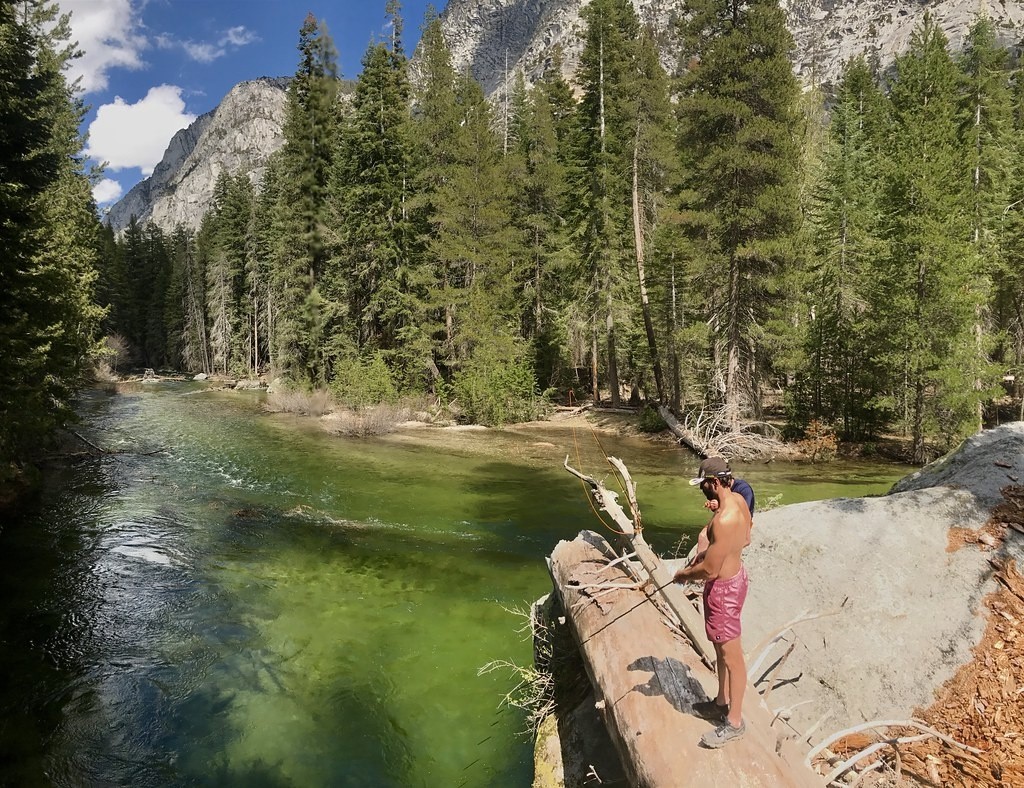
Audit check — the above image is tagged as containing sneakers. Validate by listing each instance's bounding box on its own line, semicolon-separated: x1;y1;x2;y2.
683;579;703;595
691;579;706;596
701;716;746;748
692;696;731;719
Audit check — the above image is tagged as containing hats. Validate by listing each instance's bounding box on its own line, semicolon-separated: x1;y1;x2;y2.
688;457;732;486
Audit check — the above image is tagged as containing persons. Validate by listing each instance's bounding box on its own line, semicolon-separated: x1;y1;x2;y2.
673;456;755;749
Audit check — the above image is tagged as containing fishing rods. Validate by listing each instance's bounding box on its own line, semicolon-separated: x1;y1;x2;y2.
567;387;644;536
499;563;674;692
